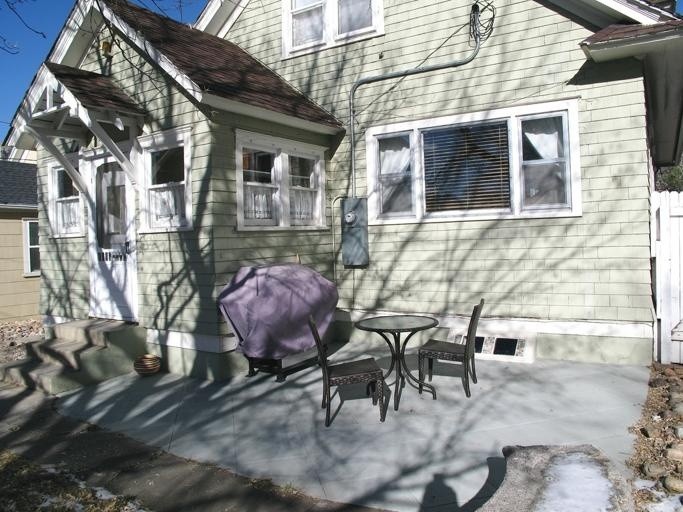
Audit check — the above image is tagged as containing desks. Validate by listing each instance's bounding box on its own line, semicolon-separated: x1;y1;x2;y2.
354;315;439;411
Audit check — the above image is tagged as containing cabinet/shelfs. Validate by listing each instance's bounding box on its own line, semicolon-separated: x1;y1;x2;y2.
247;345;328;382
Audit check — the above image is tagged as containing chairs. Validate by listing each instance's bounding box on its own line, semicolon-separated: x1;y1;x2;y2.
308;314;385;427
419;298;485;398
